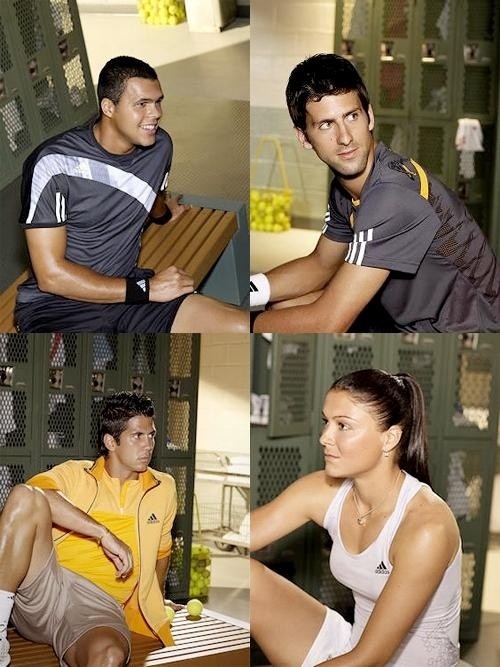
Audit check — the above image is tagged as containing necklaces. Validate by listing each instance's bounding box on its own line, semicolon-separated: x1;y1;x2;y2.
353;468;403;526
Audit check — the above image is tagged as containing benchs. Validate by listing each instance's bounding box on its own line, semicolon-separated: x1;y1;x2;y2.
0;605;250;667
0;191;250;332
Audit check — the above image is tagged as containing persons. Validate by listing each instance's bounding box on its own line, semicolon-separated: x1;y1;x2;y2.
251;367;462;666
13;57;249;332
0;392;179;667
250;51;500;334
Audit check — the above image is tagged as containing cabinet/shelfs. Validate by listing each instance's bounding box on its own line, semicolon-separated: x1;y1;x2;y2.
0;0;100;190
249;333;500;666
330;0;499;262
0;332;200;602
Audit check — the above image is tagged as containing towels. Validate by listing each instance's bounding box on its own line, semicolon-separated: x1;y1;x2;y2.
455;118;484;179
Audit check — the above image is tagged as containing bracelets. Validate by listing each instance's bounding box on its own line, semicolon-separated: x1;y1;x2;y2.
149;203;173;225
124;276;150;305
249;272;271;307
96;528;111;544
250;309;264;334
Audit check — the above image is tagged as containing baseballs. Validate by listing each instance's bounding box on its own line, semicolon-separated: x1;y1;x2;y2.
188;546;212;599
165;605;173;625
136;1;185;27
250;189;292;233
185;600;204;617
